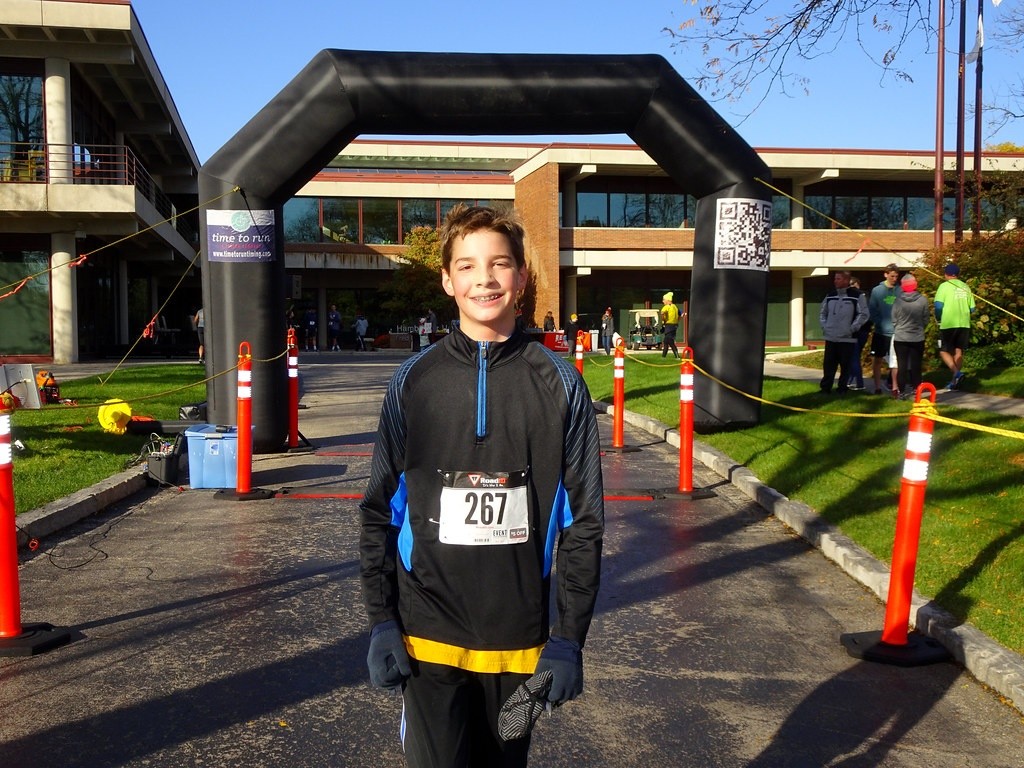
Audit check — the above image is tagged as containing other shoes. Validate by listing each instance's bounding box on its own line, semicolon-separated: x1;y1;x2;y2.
819;388;832;394
874;388;883;394
900;391;906;399
892;388;901;399
305;349;310;352
338;349;342;352
836;386;848;394
314;349;319;353
911;389;917;395
850;384;866;391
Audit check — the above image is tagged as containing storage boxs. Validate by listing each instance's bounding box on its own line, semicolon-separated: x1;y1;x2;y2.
147;426;189;486
184;424;256;489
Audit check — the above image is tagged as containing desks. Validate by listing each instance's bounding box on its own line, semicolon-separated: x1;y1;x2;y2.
434;333;448;336
540;333;569;351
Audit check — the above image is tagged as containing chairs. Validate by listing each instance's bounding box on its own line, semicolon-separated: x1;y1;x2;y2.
28;149;46;181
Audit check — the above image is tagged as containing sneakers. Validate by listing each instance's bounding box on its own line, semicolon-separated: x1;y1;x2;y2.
946;383;960;392
954;371;966;390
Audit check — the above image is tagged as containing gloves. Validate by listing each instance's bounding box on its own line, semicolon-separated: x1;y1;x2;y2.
367;621;411;688
532;639;584;710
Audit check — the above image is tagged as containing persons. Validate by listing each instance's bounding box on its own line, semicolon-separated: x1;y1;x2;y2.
564;313;580;357
155;305;205;364
418;307;437;354
543;311;558;332
285;304;369;352
359;202;605;768
602;307;614;357
660;292;682;357
934;264;976;392
819;263;928;400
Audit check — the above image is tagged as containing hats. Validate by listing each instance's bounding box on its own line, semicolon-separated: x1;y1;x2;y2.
945;263;960;277
901;274;917;292
663;292;673;302
419;317;425;323
570;313;577;320
606;307;612;315
499;669;554;739
